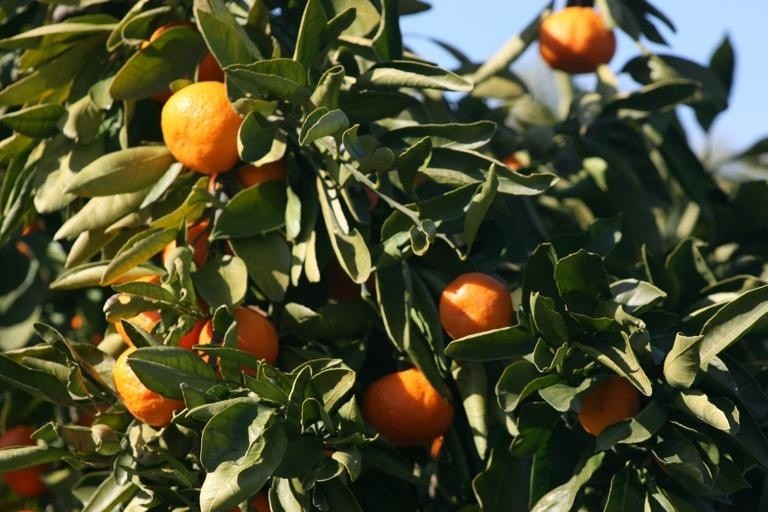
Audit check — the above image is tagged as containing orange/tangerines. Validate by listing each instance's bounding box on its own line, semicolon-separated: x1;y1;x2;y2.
0;20;522;512
576;378;640;436
539;6;616;74
